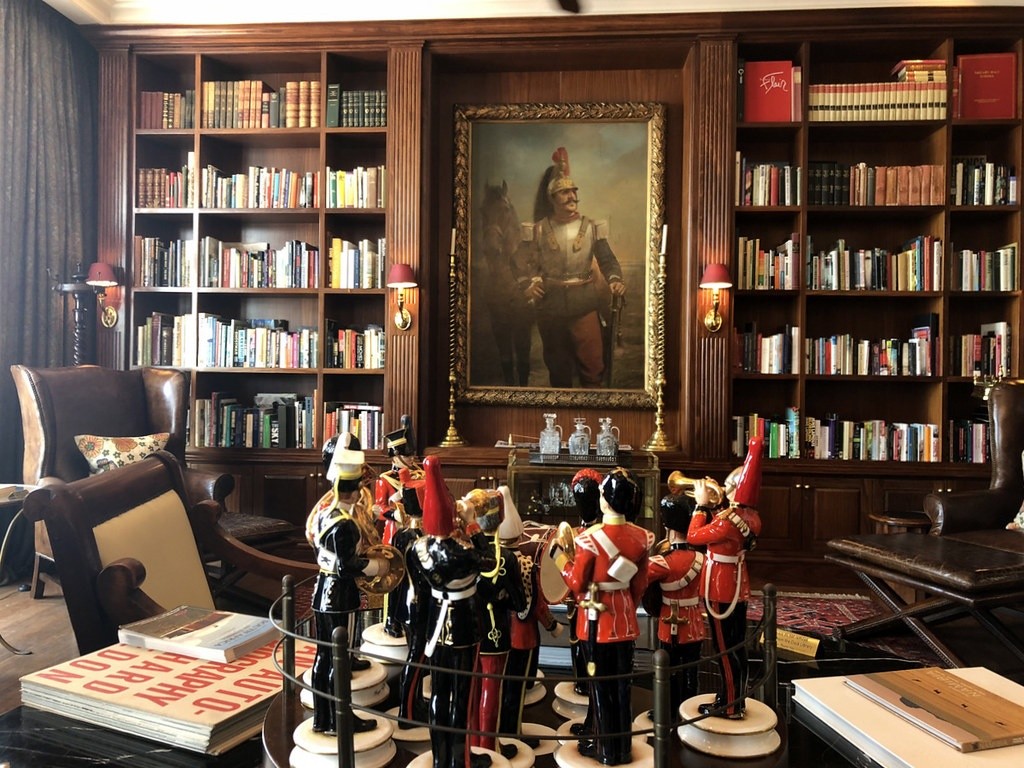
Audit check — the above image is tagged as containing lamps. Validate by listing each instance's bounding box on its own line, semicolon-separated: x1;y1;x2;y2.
87;263;120;330
698;262;734;334
386;262;418;330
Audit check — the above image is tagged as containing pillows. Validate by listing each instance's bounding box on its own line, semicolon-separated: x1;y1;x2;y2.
1006;448;1024;533
68;431;175;478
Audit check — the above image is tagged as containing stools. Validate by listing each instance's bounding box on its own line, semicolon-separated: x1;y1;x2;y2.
184;508;303;606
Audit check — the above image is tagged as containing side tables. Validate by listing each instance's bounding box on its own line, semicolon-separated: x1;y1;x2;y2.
868;510;934;613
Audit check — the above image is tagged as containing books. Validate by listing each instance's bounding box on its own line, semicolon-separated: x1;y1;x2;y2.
791;666;1024;768
135;73;386;452
731;49;1017;467
843;666;1024;754
118;604;284;663
20;633;317;755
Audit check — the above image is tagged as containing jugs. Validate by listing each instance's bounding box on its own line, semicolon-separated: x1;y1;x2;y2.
594;416;621;462
538;415;563;460
567;417;591;461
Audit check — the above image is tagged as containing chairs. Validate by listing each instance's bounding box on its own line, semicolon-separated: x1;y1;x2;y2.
11;359;236;601
925;376;1019;624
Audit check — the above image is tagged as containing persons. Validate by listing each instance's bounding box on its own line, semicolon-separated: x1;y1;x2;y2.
304;429;760;767
519;147;626;388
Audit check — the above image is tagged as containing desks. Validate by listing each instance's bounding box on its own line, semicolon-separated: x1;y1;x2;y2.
823;527;1022;670
0;650;1021;768
1;483;42;658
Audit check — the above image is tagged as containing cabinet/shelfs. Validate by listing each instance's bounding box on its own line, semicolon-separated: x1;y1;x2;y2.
185;459;252;518
729;28;1022;462
125;43;392;454
748;473;870;555
253;461;381;521
409;465;509;514
873;474;988;525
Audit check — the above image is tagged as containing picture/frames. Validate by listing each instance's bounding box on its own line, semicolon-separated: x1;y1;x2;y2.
453;96;671;409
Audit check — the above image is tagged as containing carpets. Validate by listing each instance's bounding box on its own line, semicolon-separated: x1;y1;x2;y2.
745;586;951;671
